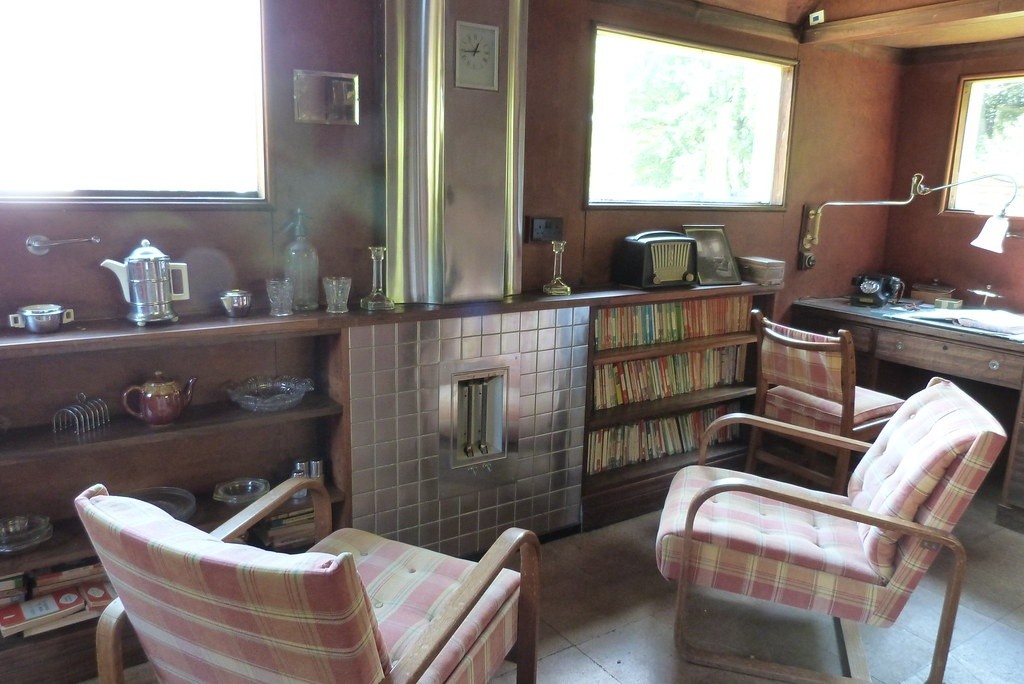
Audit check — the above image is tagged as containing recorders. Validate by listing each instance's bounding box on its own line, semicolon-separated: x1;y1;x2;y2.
620;231;698;290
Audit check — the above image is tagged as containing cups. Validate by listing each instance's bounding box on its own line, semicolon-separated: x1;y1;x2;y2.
323;277;351;313
265;277;294;316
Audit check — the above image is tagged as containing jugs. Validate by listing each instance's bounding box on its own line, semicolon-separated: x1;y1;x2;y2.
100;239;190;326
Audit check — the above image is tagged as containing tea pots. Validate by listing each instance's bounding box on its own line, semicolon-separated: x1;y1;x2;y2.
121;370;198;427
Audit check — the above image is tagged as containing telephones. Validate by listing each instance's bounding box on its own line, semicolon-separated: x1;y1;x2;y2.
851;273;900;308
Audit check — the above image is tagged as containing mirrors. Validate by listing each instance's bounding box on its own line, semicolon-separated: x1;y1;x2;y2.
293;69;360;125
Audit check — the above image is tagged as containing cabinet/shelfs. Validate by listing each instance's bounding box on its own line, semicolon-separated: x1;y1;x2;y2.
0;311;345;684
582;280;781;531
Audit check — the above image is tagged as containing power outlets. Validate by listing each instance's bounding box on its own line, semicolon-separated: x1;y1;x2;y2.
527;216;565;244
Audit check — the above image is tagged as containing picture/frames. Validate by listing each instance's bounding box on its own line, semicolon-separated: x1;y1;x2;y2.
680;223;742;287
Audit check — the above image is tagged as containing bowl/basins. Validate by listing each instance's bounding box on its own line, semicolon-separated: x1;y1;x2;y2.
0;514;53;555
225;375;314;411
212;477;270;511
116;487;196;522
218;288;252;318
9;304;74;332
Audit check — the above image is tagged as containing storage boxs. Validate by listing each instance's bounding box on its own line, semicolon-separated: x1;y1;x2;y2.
736;255;785;285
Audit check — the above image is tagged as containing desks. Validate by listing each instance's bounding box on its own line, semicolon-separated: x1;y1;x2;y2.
792;294;1024;537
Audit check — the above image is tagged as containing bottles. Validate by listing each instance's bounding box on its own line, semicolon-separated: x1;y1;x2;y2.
289;456;324;498
283;207;319;310
931;279;939;287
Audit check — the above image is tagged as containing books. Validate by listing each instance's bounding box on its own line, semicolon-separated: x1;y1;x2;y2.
587;295;752;474
0;561;118;638
915;308;1024;335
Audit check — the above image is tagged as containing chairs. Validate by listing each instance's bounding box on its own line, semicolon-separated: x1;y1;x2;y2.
75;474;541;683
655;376;1009;684
741;309;906;496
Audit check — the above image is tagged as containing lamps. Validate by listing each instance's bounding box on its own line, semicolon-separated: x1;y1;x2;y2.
796;173;1018;272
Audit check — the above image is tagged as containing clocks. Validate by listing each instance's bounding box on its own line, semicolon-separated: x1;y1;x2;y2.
454;20;502;92
